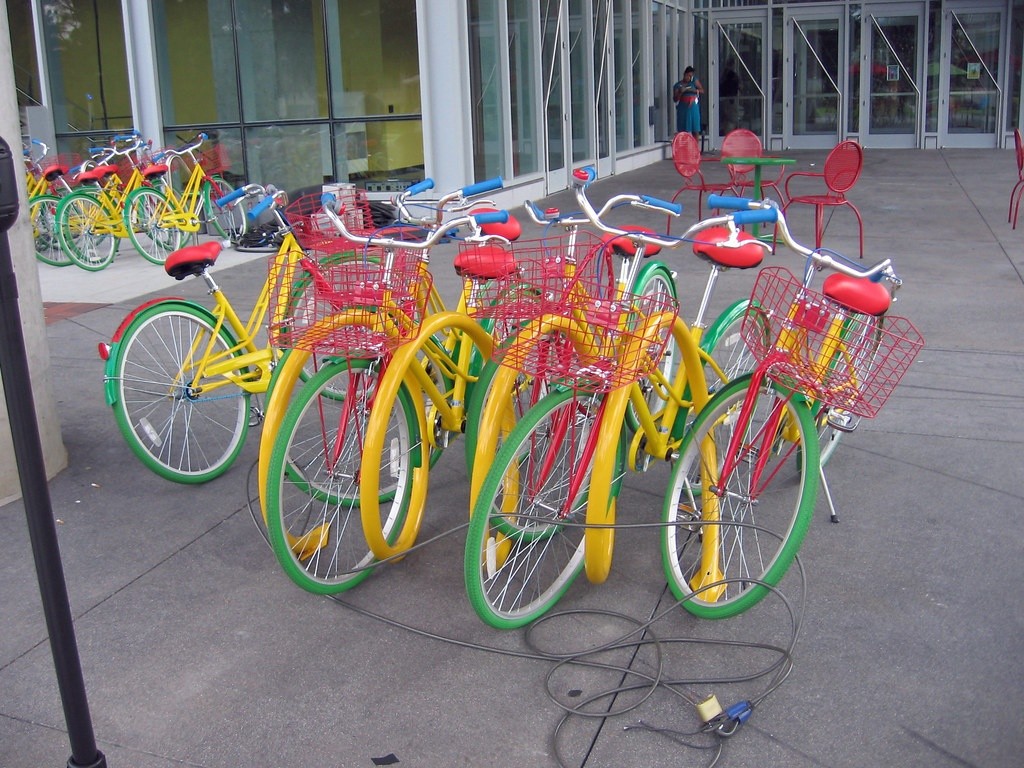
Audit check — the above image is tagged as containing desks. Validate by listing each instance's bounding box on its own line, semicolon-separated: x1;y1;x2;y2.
720;156;796;244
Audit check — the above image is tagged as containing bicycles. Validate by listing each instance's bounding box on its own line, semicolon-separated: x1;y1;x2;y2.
98;184;417;485
660;194;923;615
252;164;781;633
22;131;251;271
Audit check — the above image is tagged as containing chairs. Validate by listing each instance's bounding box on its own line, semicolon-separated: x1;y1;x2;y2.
667;131;745;236
716;129;788;232
771;141;863;265
1008;128;1024;230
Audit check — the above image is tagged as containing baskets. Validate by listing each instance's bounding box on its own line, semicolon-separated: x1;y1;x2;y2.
42;153;82;187
458;229;680;394
267;190;433;359
99;145;180;186
183;143;231;176
740;267;926;418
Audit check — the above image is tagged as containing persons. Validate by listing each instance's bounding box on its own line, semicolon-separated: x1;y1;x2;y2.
673;66;705;144
719;57;753;136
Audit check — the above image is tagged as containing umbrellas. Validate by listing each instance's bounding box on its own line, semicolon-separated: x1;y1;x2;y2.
927;62;968;76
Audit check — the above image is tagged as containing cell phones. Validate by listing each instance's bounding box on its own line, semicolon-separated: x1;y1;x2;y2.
691;76;693;82
